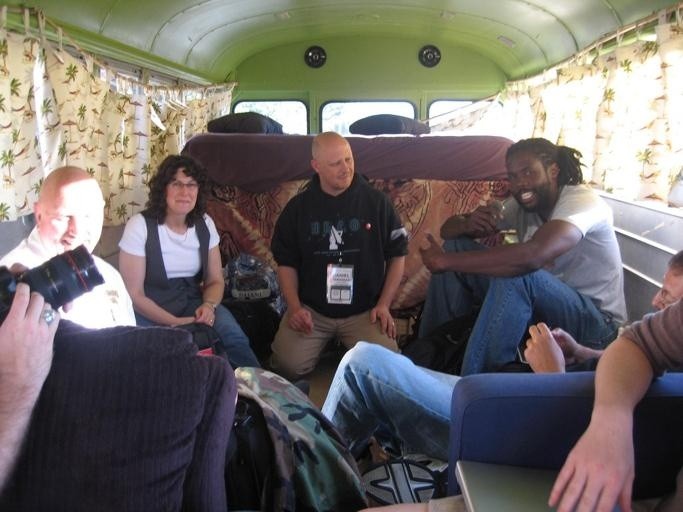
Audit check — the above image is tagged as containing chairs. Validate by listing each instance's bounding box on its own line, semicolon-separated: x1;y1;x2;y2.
1;318;240;512
447;372;682;497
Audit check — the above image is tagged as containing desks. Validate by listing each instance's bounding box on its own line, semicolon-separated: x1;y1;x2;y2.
453;460;662;512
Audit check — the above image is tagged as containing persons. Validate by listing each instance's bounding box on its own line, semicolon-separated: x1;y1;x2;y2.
398;137;629;377
1;262;73;493
117;154;261;369
321;250;683;463
261;130;409;377
1;165;137;330
547;299;683;512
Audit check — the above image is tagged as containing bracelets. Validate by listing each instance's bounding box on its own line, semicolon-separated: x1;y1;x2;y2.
202;301;216;312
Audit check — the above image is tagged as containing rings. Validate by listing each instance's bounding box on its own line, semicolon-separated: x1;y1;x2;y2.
392;321;395;326
44;309;55;324
210;319;213;323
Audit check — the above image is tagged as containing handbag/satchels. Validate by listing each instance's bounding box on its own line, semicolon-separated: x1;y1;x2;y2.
223;299;280;361
224;367;368;510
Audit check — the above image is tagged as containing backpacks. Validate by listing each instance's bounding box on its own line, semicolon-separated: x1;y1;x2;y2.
222;254;281;314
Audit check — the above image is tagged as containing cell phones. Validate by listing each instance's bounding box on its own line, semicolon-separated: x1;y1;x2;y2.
490;200;505;220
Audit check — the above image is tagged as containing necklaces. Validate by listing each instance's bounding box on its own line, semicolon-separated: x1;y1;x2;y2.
165;219;188;242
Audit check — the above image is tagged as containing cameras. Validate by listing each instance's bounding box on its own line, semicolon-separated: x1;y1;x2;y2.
0;243;106;326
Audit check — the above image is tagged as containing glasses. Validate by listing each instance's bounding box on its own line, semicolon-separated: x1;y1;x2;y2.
173;180;200;191
